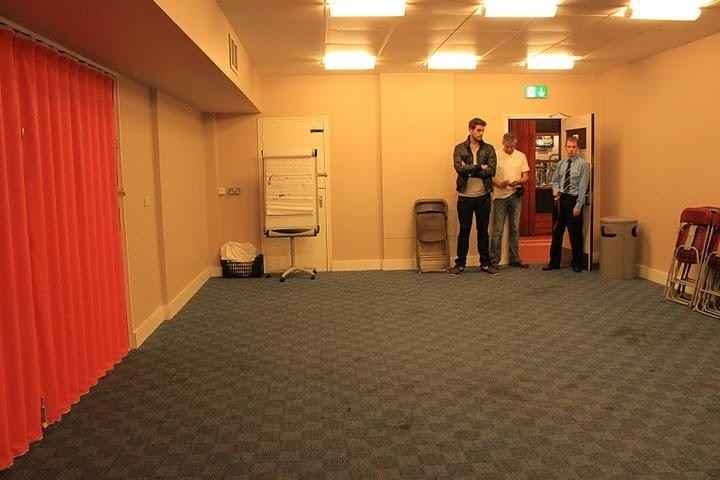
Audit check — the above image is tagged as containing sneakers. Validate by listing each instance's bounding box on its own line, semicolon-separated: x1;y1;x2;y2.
449;266;465;275
571;264;581;272
508;261;528;268
542;264;560;271
481;263;500;274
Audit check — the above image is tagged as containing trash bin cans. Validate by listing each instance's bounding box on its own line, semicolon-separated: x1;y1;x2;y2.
600;217;638;280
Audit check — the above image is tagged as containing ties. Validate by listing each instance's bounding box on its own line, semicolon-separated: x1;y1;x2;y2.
563;160;572;193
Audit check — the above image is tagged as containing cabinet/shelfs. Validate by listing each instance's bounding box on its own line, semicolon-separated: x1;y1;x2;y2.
507;117;560;235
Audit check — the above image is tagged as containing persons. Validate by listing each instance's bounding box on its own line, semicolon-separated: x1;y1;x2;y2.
490;134;530;268
449;118;497;274
542;135;590;272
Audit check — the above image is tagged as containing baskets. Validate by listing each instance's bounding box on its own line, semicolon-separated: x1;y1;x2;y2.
221;254;263;279
